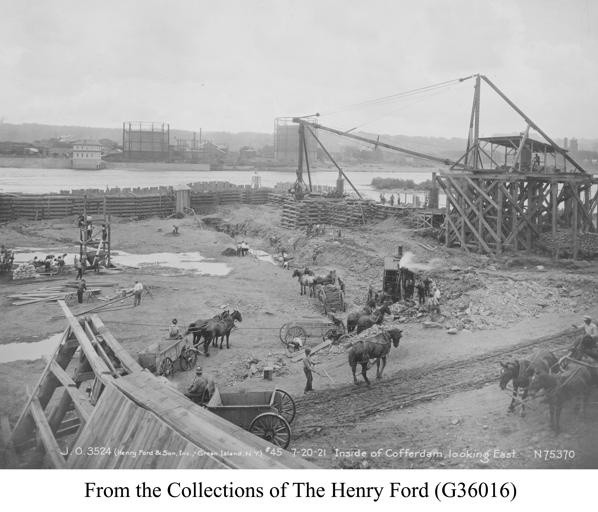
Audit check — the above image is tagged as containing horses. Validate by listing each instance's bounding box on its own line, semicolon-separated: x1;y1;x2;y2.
498;349;559;418
527;364;593;435
348;327;403;386
185;309;230;350
292;268;316;298
357;302;392;335
347;302;376;332
202;310;243;357
303;267;323;292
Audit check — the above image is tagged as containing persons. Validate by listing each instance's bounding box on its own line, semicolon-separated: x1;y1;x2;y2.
571;315;598;350
416;281;426;304
379;193;400;206
237;240;248;257
74;213;107;280
133;280;143;307
184;366;208;394
367;294;378;309
423;276;432;296
302;347;321;394
0;245;7;263
169;318;181;338
33;256;65;273
77;279;88;303
533;154;540;166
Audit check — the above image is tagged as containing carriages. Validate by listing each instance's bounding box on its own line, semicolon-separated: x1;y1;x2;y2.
279;288;393;350
140;303;242;377
292;264;348;317
494;332;596;436
